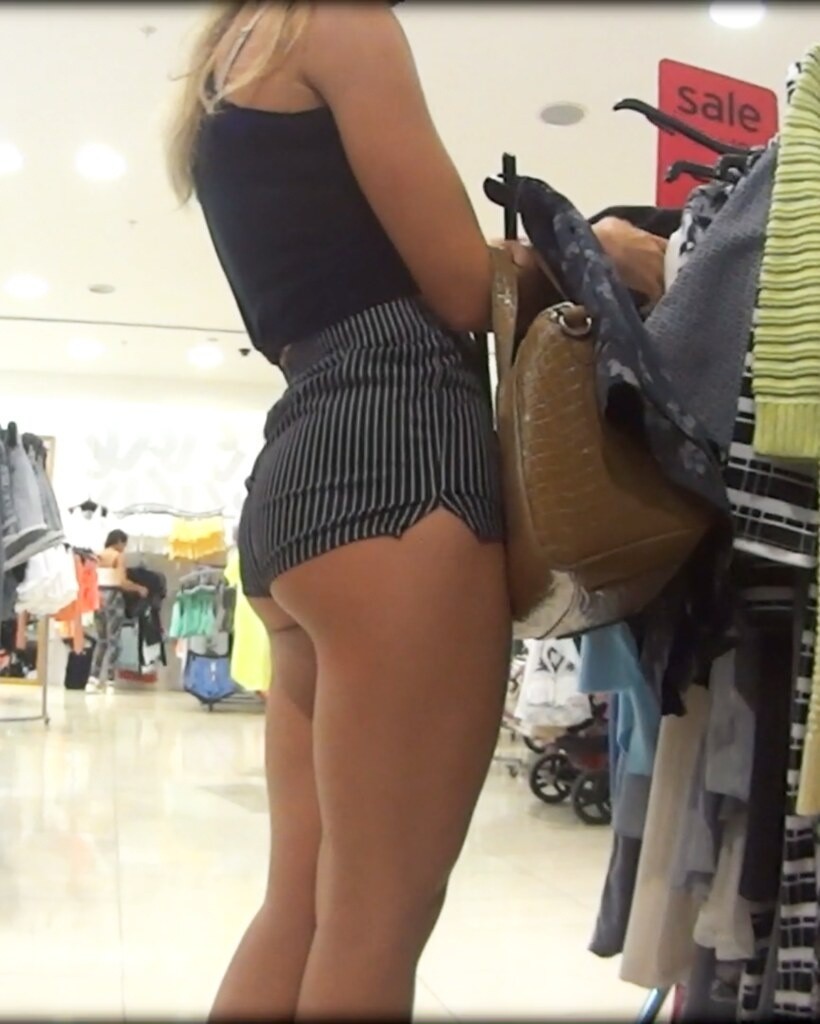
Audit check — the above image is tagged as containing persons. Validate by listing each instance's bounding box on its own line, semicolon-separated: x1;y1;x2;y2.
84;529;149;693
156;2;663;1024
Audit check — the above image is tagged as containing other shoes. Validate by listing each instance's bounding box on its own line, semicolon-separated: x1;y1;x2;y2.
84;676;103;693
106;680;115;694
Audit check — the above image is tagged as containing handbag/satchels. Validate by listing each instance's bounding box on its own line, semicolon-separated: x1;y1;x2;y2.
487;236;704;638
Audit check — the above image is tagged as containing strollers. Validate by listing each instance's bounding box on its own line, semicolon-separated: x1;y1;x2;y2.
526;631;619;827
